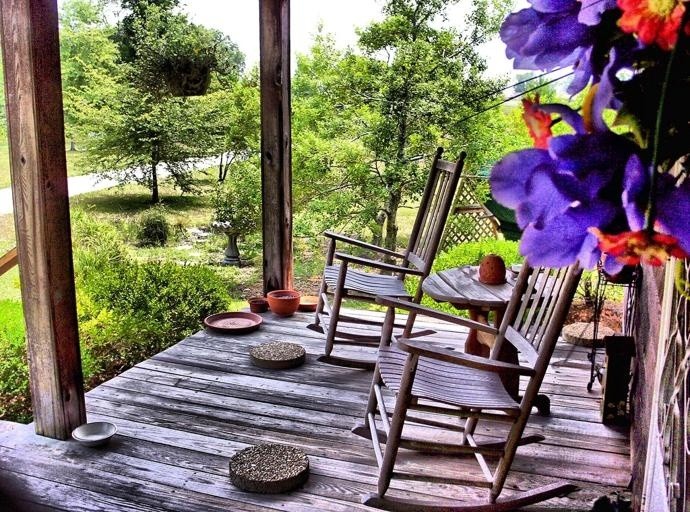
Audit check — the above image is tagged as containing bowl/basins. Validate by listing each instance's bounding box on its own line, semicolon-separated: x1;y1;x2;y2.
298;295;319;311
70;420;118;446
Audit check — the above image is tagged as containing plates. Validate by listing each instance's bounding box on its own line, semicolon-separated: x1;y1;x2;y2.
204;311;262;330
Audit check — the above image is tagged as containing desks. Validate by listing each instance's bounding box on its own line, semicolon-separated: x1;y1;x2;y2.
421;265;559;419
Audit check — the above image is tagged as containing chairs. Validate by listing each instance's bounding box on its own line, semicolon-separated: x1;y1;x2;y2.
305;147;467;370
351;262;584;512
